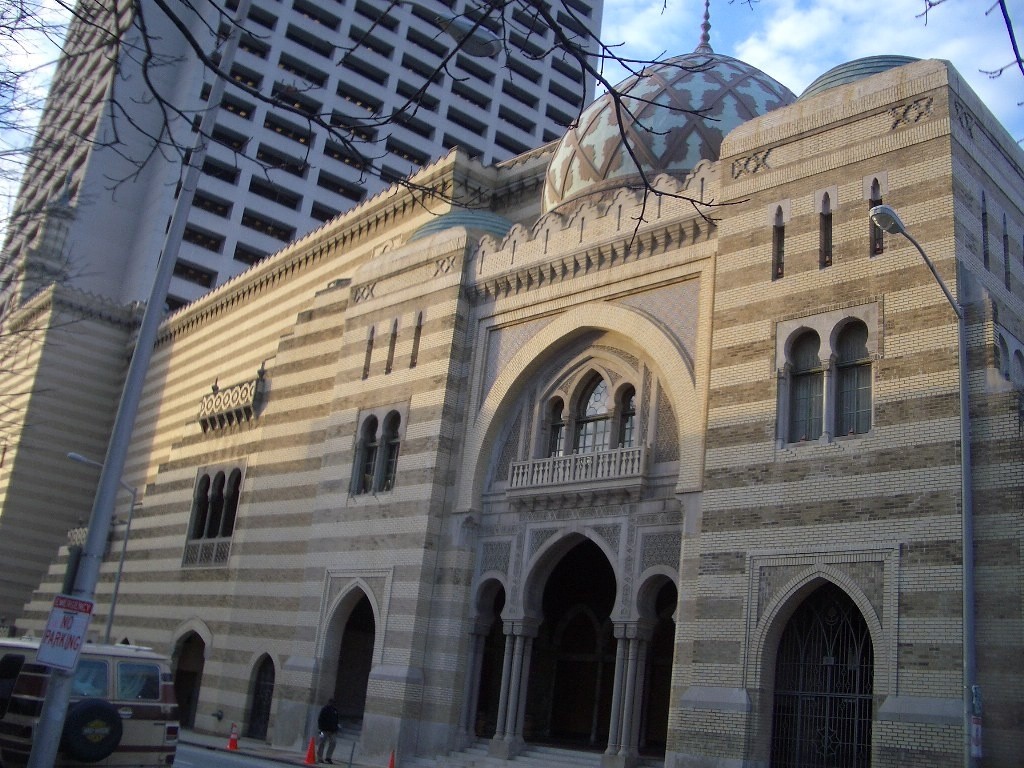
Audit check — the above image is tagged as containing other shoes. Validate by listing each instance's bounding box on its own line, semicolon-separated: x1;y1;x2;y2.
325;758;333;764
318;760;324;763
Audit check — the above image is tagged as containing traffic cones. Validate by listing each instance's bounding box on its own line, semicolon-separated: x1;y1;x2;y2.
386;749;395;768
305;737;316;765
225;722;238;750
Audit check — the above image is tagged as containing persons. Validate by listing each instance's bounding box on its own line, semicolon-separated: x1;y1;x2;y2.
316;699;343;765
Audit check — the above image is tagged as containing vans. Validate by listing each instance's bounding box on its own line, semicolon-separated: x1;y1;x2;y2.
0;632;181;768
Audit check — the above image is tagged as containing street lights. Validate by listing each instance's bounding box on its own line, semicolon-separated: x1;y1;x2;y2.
66;451;142;644
868;204;986;768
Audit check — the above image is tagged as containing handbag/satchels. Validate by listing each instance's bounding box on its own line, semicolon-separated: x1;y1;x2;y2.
319;731;324;739
